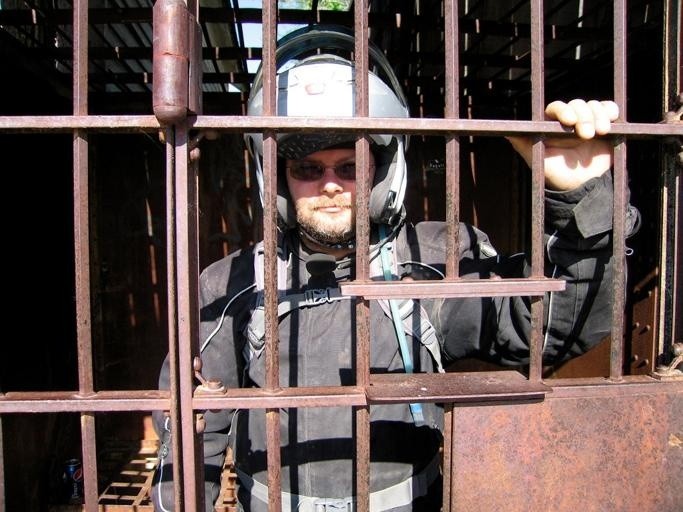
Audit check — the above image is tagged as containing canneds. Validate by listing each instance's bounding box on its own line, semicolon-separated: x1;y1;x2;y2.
62;457;82;500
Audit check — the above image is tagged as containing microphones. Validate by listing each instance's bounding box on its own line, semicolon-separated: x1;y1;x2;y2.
306;203;407;277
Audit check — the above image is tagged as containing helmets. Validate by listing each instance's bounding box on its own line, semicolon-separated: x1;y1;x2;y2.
243;53;412;233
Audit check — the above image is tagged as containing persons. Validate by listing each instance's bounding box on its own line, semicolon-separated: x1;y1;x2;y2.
148;25;641;511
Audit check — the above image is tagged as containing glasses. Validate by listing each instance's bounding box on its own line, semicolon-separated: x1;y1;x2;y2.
285;157;353;181
